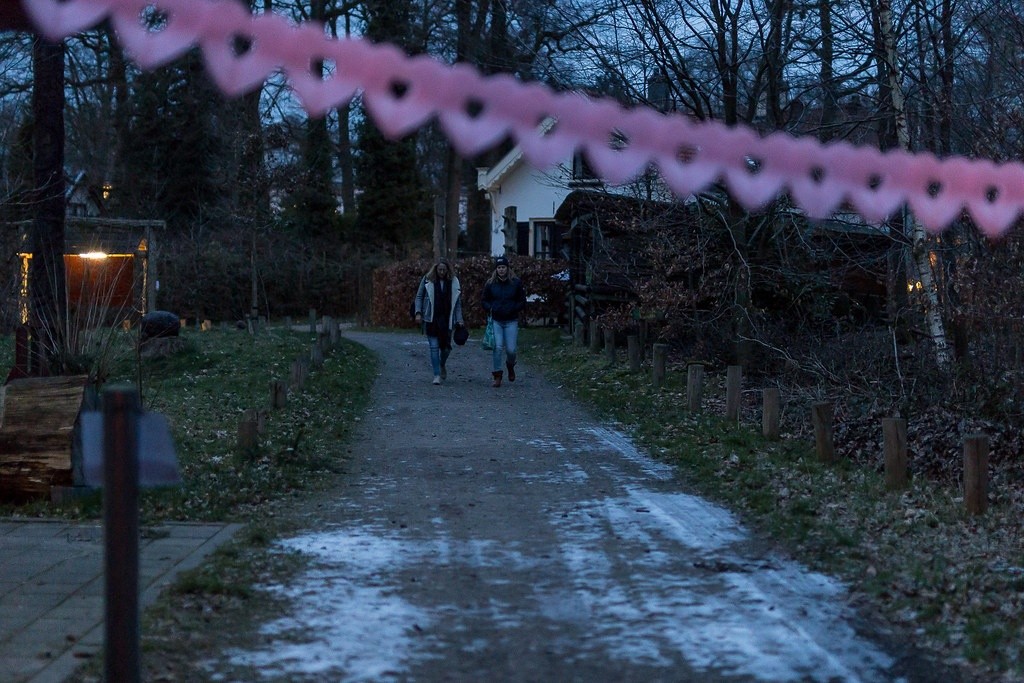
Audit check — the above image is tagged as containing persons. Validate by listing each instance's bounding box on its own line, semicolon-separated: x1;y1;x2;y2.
482;257;526;387
414;258;464;384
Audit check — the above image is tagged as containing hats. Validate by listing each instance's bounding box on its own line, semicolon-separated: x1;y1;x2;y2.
494;258;509;270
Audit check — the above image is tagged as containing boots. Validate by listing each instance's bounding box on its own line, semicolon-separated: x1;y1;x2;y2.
506;361;516;381
492;369;503;387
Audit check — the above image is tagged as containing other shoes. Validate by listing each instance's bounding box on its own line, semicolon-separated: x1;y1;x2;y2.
433;376;442;385
441;370;447;379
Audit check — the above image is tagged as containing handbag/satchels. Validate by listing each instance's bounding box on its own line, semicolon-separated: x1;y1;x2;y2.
454;325;469;346
482;316;495;351
